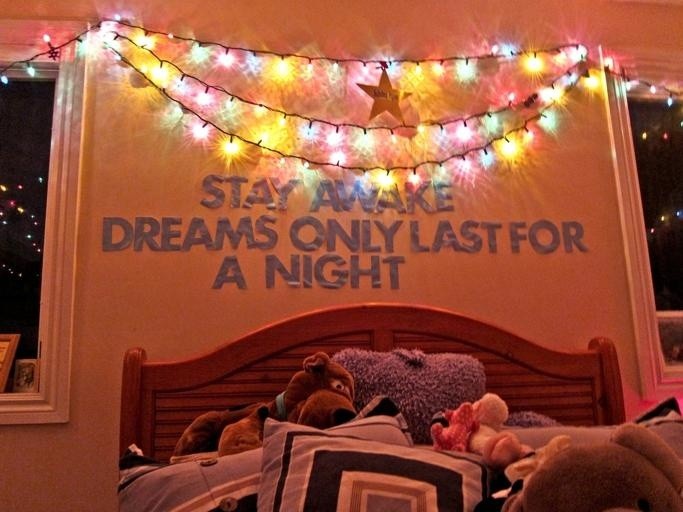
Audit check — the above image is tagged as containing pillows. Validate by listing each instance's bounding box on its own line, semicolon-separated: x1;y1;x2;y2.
118;397;486;512
629;398;682;464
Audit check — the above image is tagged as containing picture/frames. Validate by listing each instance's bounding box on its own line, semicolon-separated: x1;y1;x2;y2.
655;310;682;372
0;332;40;394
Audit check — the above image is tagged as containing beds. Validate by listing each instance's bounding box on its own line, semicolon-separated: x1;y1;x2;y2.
116;300;682;511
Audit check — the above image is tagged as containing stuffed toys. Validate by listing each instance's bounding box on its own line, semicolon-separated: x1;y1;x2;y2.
428;400;481;453
168;348;355;465
501;420;682;512
468;391;536;468
